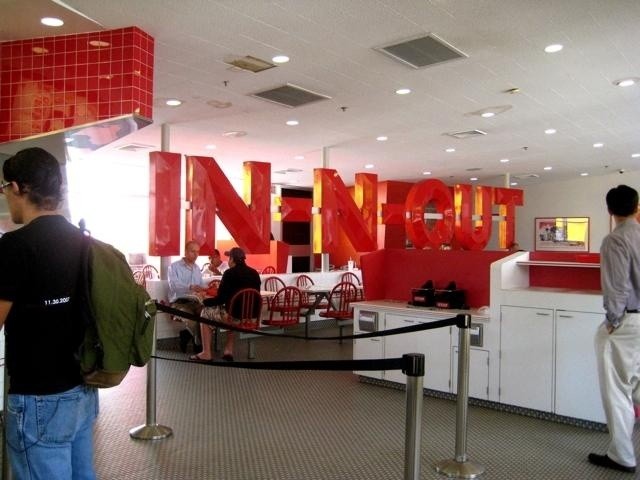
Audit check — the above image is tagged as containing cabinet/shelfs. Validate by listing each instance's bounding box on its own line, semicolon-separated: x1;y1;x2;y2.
489;250;639;426
350;299;490;401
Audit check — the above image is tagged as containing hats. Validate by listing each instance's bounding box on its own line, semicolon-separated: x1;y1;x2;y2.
224;248;245;258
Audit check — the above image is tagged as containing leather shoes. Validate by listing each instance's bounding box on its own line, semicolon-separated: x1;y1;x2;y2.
587;453;636;473
193;342;203;354
179;330;187;353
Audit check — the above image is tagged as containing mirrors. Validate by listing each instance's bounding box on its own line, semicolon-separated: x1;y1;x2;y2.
535;217;590;253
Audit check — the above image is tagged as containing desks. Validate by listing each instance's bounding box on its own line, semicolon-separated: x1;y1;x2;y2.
193;291;278;358
296;286;363;343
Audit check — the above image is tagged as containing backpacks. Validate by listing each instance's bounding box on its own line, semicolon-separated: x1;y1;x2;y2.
80;225;157;388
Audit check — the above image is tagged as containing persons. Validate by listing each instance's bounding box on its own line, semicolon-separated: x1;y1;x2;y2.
167;242;204;353
189;247;262;362
586;183;640;476
0;147;101;480
201;248;230;276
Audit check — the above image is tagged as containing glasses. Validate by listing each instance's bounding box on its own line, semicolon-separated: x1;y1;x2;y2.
0;180;12;194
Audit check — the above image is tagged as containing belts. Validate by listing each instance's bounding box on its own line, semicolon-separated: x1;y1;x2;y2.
627;310;638;313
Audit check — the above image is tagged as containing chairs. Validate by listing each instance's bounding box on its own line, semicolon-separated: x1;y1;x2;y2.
261;266;275;274
133;271;146;291
228;288;263;331
262;286;302;326
265;277;296;312
208;280;222;290
143;266;160;290
319;282;357;319
201;263;216;276
296;275;331;309
339;272;363;302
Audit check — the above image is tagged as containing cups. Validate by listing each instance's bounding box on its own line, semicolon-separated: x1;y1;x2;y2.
206;263;210;268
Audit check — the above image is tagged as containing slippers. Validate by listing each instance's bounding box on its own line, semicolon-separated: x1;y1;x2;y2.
188;355;213;362
221;352;234;361
